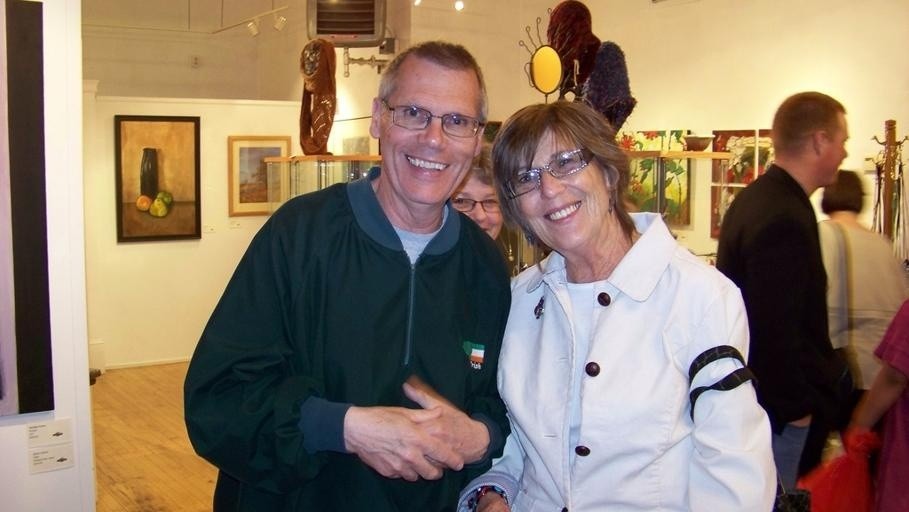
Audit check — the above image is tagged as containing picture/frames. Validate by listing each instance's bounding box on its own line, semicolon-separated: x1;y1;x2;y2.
229;136;291;216
114;115;201;241
711;129;775;239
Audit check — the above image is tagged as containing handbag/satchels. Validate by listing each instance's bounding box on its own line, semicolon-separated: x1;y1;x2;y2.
834;348;863;394
773;489;810;512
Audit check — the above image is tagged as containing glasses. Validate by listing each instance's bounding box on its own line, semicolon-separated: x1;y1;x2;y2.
503;149;595;199
451;199;500;212
385;101;485;138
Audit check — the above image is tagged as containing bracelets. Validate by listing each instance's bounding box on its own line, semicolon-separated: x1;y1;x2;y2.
469;485;511;509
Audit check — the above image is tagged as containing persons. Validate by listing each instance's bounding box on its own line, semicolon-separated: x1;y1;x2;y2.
453;98;779;512
182;41;512;512
446;142;506;242
835;295;909;511
714;90;855;511
817;165;909;472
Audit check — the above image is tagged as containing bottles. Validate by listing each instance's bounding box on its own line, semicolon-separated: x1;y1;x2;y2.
140;148;158;198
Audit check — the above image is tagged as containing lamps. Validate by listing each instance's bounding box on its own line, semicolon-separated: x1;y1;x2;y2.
413;0;420;7
272;12;287;32
455;1;464;11
247;19;263;37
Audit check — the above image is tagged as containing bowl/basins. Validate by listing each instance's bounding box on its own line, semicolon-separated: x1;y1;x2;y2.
682;134;716;151
